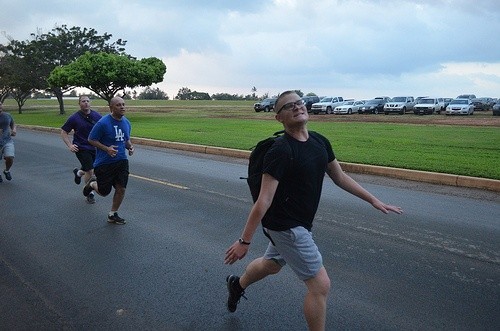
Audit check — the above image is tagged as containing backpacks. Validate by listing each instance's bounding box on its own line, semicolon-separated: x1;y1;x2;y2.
239;129;289;247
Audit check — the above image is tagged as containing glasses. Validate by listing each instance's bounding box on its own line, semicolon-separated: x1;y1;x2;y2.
275;99;306;114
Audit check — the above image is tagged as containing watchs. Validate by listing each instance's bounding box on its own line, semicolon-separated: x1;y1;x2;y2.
238;237;250;245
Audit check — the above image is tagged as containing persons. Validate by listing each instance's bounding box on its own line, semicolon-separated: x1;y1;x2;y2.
0;103;16;181
224;91;404;331
82;97;135;225
60;95;102;203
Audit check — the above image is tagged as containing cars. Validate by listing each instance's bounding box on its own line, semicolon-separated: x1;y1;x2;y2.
253;98;278;112
492;99;500;116
357;99;385;115
446;98;474;115
333;101;364;115
456;95;497;111
413;97;441;115
442;97;453;107
383;96;417;115
301;96;320;110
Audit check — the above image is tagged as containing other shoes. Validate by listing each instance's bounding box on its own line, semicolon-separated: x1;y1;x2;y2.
0;169;13;183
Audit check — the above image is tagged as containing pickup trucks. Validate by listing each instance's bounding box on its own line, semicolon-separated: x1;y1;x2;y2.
311;96;345;114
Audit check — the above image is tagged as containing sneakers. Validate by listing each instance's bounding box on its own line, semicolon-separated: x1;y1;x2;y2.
225;274;248;313
72;167;82;185
107;211;126;225
87;193;97;203
82;176;97;197
